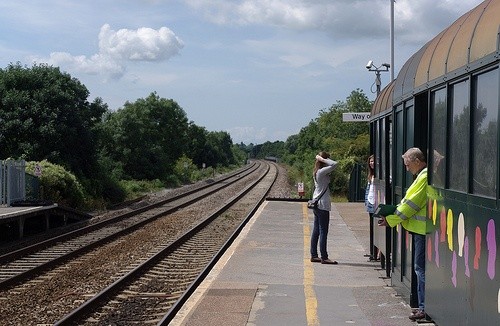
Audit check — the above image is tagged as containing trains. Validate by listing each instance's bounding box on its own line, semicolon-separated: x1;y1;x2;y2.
364;0;500;326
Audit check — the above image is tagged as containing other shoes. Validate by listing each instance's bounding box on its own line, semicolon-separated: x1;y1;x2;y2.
409;309;426;319
322;259;338;264
311;257;321;262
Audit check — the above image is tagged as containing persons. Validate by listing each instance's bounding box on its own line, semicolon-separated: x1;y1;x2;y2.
377;147;429;320
364;154;380;260
310;152;339;264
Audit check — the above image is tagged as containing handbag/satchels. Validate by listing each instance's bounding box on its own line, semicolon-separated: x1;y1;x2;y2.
307;200;317;210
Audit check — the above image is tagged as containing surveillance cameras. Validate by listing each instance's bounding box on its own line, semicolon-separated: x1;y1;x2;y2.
366;60;373;68
